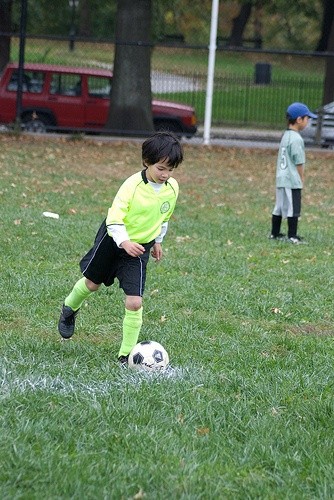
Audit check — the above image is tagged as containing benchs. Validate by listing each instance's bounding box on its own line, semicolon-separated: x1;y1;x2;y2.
217;37;263;48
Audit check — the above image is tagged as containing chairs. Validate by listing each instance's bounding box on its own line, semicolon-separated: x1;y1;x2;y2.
76;80;82;96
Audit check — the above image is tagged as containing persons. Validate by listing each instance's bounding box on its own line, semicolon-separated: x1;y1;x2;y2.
269;103;318;242
58;132;183;366
10;73;28;91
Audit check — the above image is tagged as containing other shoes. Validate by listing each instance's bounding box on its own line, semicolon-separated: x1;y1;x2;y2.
270;233;283;240
286;236;308;245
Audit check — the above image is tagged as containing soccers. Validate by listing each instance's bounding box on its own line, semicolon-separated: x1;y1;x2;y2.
128;339;169;370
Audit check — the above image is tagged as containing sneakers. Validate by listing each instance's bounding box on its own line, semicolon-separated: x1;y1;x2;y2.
58;304;78;338
117;353;129;366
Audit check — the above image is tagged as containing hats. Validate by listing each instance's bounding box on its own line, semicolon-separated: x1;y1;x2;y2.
287;103;319;120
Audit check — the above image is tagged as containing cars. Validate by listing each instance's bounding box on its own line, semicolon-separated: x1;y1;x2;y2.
0;59;196;140
302;99;334;148
